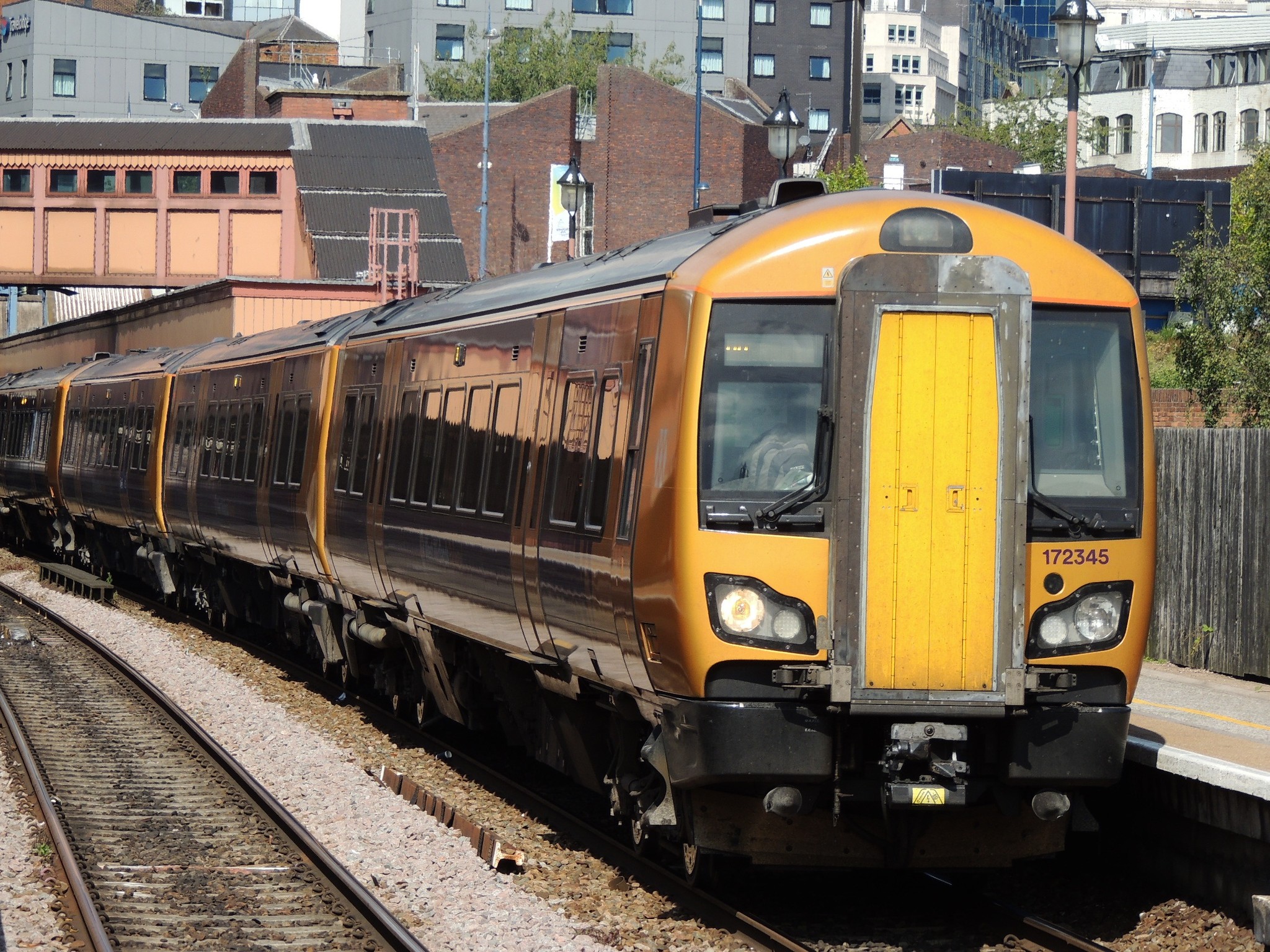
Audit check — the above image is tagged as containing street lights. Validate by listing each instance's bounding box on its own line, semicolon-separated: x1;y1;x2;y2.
1049;0;1105;240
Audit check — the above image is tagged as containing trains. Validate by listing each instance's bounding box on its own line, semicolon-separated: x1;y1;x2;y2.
0;178;1160;887
